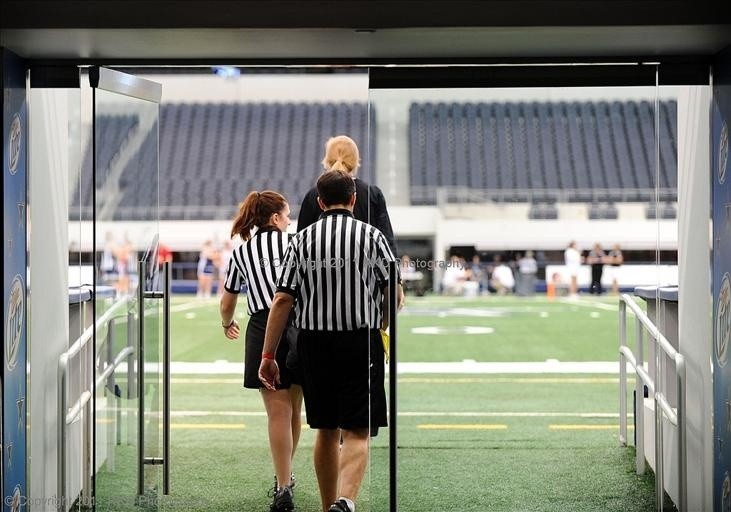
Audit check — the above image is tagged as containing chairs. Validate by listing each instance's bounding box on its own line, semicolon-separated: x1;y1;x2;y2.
69;101;677;299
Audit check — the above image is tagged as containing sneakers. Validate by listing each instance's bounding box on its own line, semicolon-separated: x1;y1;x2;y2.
328;498;354;512
269;473;295;512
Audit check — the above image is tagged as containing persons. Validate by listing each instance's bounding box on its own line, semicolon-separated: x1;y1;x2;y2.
216;240;238;296
158;242;174;263
585;242;608;294
607;242;625;266
295;135;404;459
221;191;308;511
114;238;131;295
197;239;220;296
563;241;586;297
99;232;118;285
400;255;424;296
443;246;539;296
257;169;401;511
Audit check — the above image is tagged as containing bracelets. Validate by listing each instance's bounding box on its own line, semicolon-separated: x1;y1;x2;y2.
222;319;235;328
263;352;279;360
